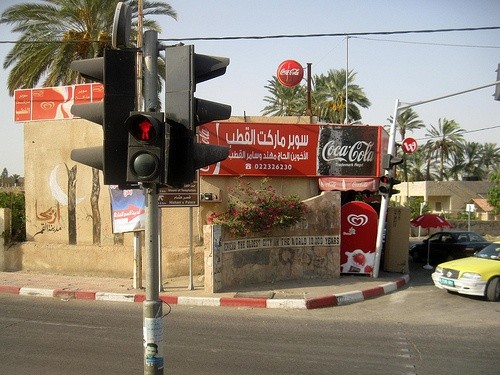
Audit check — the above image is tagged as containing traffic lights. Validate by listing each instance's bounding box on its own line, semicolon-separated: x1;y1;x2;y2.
70;50;139;188
166;44;232;194
127;146;166;187
125;109;166;148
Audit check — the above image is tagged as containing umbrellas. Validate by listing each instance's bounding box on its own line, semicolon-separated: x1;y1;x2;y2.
410;212;452;269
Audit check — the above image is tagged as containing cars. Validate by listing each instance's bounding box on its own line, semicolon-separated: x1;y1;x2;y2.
409;231;492;271
432;241;500;303
